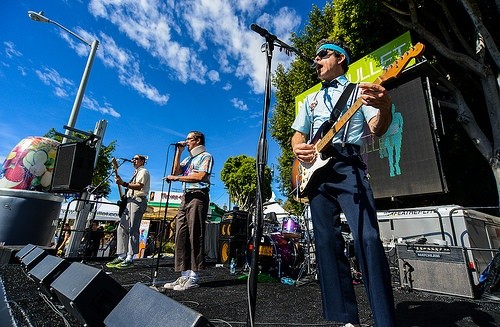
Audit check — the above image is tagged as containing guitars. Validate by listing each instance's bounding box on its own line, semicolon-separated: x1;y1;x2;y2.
109;156;128;218
290;41;425;198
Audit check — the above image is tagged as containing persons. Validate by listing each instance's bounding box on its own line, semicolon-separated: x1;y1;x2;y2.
55;220;117;257
164;131;214;290
106;154;150;269
291;39;395;327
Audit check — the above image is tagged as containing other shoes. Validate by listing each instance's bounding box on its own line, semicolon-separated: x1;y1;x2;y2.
164;275;199;291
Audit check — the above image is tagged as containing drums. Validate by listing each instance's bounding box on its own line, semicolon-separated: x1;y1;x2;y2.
281;216;306;240
245;231;302;275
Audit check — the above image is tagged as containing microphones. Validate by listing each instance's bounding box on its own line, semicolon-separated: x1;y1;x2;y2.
171;143;186;147
303;55;318;69
121;158;134;163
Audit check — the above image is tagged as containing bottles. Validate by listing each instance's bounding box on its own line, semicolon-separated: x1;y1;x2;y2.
280;276;296;285
230;257;236;274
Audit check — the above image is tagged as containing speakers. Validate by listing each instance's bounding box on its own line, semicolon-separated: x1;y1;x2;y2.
217;219;249;266
49;142;97;193
396;243;476;299
16;244;216;327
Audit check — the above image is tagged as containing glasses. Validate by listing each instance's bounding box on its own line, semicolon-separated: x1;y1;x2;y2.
185;137;193;140
312;48;339;58
132;157;140;160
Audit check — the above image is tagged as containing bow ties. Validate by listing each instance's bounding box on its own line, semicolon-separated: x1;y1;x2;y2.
320;80;338;90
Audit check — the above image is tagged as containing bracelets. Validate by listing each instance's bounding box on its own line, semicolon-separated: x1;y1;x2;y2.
176;176;179;180
123;182;129;187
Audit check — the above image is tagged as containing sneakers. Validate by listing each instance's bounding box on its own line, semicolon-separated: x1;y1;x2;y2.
106;256;124;268
114;258;134;269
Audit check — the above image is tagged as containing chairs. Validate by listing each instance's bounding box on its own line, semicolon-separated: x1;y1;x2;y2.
269;233;304;279
